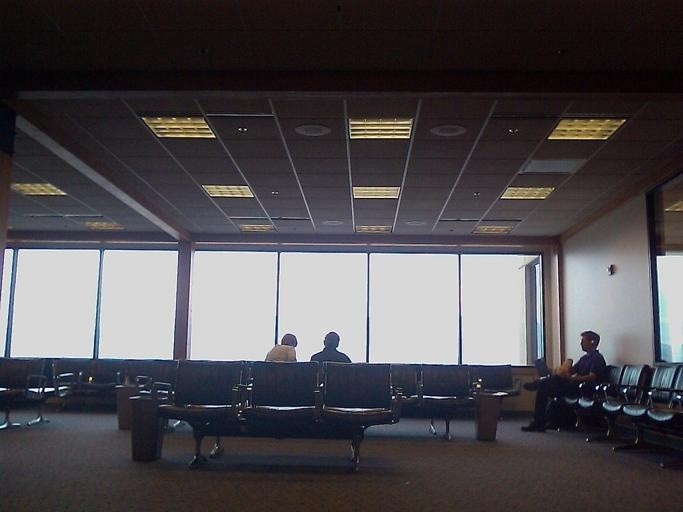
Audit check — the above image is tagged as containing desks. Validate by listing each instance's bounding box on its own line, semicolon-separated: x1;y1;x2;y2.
114;383;146;430
129;395;169;461
475;391;510;442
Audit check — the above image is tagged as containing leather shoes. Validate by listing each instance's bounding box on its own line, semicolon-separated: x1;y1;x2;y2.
524;381;536;391
522;425;545;432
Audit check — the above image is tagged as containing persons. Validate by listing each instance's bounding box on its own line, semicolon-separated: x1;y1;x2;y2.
264;333;298;362
520;330;608;433
309;331;353;363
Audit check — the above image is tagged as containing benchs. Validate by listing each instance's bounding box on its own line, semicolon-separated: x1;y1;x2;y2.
157;361;403;473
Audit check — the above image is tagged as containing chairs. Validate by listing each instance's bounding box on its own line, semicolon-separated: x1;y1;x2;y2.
476;365;522;422
422;365;476;442
553;364;683;469
0;358;178;431
391;364;419;407
